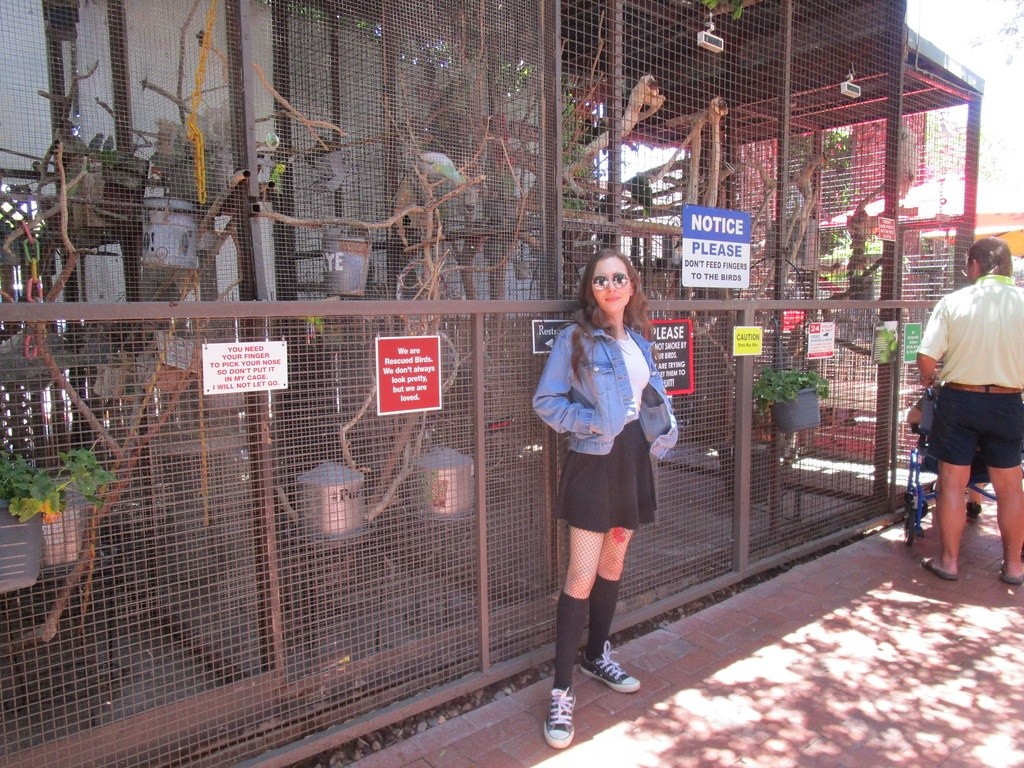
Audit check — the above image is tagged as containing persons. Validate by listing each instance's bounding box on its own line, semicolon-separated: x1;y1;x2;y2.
533;249;678;748
918;238;1024;584
909;395;983;519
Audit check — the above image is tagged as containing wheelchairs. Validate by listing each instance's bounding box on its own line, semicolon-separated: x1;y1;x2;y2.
903;383;997;547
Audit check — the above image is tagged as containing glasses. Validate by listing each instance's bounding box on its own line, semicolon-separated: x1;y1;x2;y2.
962;264;971;277
591;273;630;290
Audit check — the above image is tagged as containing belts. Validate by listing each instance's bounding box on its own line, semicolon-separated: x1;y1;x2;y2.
942;383;1024;394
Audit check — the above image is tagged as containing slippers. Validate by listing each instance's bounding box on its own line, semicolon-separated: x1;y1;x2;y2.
921;556;958;581
999;557;1024;584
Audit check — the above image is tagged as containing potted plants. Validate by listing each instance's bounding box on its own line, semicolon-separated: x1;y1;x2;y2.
0;448;118;595
751;365;830;435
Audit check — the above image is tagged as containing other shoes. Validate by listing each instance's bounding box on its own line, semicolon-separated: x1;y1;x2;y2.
966;501;982;522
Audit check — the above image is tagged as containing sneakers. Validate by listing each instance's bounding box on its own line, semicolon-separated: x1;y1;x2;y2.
580;640;641;693
544;687;576;749
